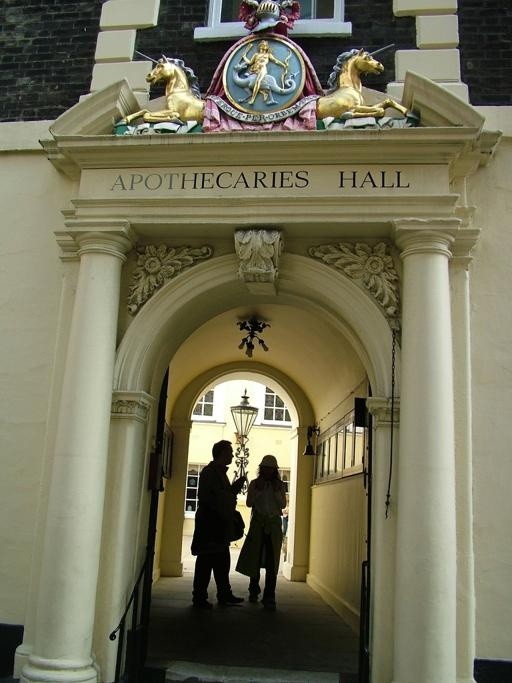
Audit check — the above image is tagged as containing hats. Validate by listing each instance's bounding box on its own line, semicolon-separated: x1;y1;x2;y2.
257;454;279;469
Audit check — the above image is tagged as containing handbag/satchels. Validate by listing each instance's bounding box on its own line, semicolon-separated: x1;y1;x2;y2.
229;510;245;543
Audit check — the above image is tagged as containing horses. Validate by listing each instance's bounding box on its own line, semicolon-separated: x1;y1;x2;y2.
315;41;422;122
110;47;207;128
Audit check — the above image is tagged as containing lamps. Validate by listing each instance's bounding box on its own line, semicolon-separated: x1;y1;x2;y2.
303;426;319;455
236;317;270;358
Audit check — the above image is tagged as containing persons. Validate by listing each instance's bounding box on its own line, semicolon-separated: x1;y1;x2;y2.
243;40;286;105
193;440;247;608
235;455;286;614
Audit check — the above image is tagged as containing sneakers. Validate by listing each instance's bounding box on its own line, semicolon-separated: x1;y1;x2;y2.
247;595;259;602
216;591;245;605
192;600;215;611
260;596;276;611
